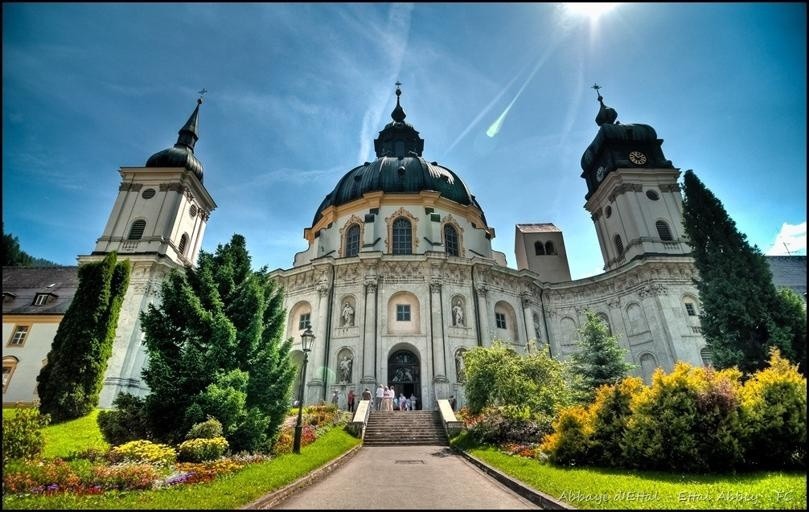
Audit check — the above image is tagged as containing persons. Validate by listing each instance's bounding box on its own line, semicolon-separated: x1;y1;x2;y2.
340;355;352;380
376;384;417;411
362;388;371;400
452;300;464;326
332;390;339;404
342;302;354;326
348;390;354;412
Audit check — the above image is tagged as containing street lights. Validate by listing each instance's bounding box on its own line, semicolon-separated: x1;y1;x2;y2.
293;325;317;456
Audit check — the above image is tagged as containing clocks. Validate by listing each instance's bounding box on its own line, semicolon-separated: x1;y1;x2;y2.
595;164;608;182
629;151;647;165
